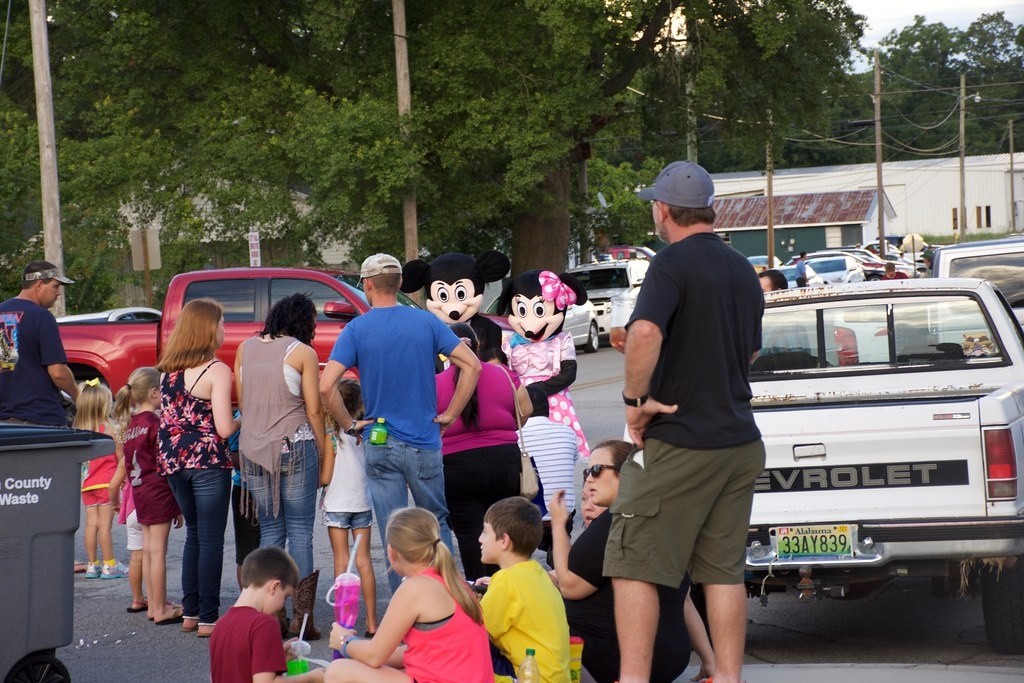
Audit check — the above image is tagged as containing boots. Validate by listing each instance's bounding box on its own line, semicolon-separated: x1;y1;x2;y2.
288;570;321;641
276;595;290;640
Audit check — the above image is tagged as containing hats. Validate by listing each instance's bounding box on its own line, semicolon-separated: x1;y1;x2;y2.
21;267;75;286
355;254;402;287
920;250;933;258
637;161;714;208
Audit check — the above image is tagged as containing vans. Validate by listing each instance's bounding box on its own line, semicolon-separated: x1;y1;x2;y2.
593;245;657;262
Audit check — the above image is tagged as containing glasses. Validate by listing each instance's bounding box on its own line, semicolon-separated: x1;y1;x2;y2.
582;464;616;480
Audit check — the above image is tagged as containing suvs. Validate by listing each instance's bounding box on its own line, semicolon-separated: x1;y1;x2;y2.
565;258;651;348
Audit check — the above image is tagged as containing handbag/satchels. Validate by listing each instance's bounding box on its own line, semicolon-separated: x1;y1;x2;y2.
520;451;539;501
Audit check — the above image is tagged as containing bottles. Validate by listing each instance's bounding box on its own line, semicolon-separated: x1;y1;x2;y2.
332;573;361;657
516;649;540;683
369;417;388;446
286;641;310;675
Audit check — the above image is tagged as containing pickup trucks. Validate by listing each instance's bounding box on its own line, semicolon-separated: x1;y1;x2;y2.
619;276;1024;657
58;266;424;430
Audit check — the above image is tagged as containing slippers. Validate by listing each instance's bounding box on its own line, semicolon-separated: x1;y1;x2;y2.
147;604;183;621
154;605;186;625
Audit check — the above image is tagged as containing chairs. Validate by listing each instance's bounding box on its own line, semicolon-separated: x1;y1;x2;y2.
749;324;816;371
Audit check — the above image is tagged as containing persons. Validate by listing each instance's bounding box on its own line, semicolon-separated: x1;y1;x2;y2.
209;546;327;683
152;297;244;635
234;292;327;640
71;378;129;578
321;378;377;638
228;410;259;591
109;456;171;611
0;260;88;575
436;321;714;683
111;366;184;624
602;160;767;683
757;242;934;292
319;253;482;595
326;508;495;683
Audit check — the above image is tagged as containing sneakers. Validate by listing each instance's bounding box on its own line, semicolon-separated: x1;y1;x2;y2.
100;561;129;579
85;560;102;578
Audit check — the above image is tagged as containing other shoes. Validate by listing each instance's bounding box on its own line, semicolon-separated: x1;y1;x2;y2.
127;600;148;613
365;631;376;639
180;615;200;632
74;560;86;573
690;665;710;681
198;617;221;638
146;599;175;610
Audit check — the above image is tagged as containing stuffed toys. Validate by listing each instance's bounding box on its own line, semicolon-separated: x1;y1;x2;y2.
397;250;591;462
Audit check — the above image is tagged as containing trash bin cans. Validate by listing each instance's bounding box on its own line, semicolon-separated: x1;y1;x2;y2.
0;423;116;683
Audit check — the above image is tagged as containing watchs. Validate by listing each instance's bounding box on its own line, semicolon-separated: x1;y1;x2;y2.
343;419;356;435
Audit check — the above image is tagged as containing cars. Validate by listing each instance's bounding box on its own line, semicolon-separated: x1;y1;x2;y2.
746;233;1024;363
55;306;163;323
483;290;600;354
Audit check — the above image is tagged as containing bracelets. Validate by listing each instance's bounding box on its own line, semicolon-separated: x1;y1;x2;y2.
621;389;649;406
337;634;359;659
